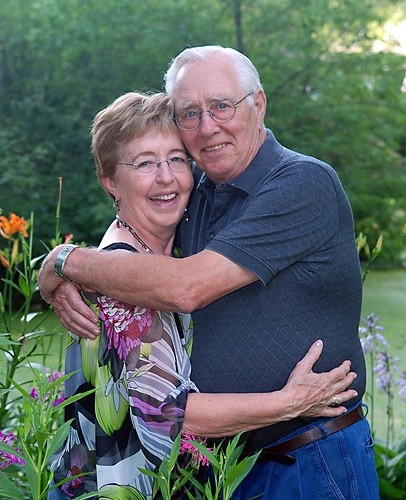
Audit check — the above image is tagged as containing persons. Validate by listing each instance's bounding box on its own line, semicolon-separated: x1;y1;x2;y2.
46;94;358;500
36;46;381;500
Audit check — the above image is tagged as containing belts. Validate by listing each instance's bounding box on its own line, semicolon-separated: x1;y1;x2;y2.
240;406;365;467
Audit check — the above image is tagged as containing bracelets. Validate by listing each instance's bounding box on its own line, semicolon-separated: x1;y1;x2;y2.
53;244;81;279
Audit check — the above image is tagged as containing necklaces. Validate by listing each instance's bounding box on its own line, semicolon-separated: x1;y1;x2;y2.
115;215;154;254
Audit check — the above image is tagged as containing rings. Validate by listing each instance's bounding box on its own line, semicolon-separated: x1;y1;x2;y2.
332;396;342;407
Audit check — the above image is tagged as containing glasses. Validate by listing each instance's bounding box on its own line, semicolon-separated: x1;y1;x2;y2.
113;153;193;176
172;91;255;131
